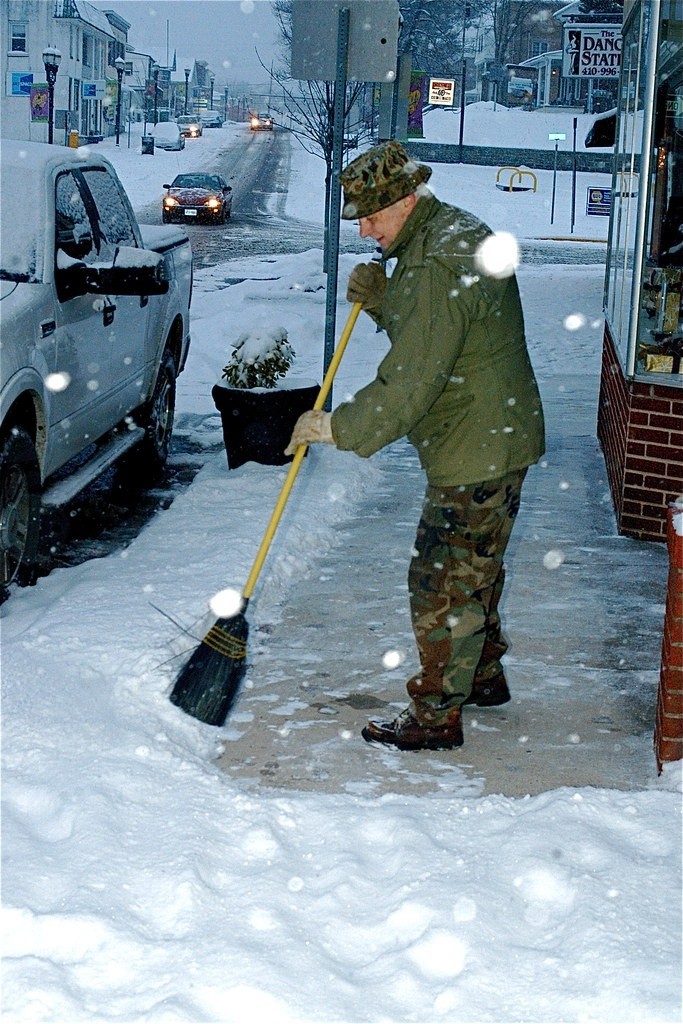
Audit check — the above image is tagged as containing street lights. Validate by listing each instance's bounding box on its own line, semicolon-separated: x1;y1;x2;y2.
224;85;229;121
237;97;240;122
42;43;62;144
153;63;160;126
113;56;126;146
209;76;215;110
184;66;191;116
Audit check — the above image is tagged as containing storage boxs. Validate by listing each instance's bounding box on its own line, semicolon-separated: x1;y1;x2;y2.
640;262;683;375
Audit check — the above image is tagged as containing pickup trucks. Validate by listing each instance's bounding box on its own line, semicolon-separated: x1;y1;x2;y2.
0;139;193;590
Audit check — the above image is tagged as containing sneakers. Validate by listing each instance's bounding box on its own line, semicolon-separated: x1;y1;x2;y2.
462;673;512;706
361;708;464;749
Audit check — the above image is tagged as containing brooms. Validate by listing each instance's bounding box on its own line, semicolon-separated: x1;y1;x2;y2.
149;246;383;728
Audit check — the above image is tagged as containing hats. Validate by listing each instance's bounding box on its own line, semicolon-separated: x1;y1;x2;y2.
338;140;433;219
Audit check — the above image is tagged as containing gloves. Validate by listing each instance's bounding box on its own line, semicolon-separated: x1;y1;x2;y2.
346;263;390;311
284;408;334;457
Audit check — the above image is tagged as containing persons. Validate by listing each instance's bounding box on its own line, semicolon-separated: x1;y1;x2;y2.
284;141;545;752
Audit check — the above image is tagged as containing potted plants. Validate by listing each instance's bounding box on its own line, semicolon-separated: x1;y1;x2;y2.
211;326;320;471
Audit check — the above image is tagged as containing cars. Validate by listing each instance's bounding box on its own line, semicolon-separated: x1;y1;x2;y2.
176;115;202;138
161;171;234;225
250;113;275;130
197;110;223;128
146;122;186;151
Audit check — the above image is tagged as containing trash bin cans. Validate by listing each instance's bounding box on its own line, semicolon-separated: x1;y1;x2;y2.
141;136;155;156
